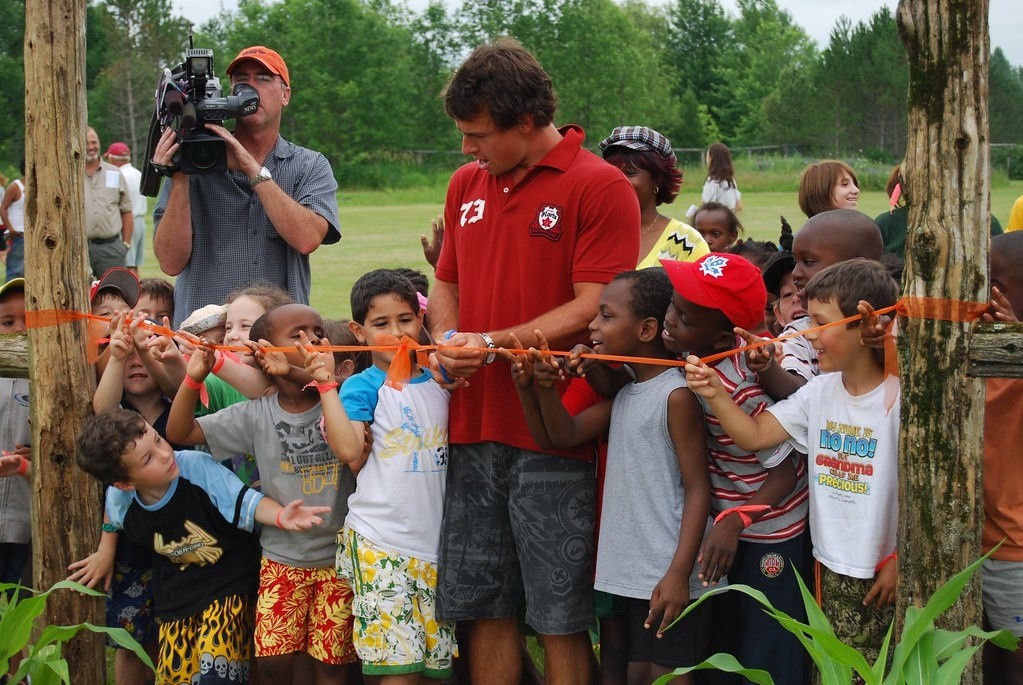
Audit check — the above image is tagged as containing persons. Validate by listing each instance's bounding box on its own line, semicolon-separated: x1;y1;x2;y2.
426;41;639;685
148;45;343;337
0;127;1023;685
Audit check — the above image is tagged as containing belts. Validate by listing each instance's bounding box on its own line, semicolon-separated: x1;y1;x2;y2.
88;235;118;244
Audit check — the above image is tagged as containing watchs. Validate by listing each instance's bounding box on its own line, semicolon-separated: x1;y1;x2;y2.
478;332;496;367
248;166;271;187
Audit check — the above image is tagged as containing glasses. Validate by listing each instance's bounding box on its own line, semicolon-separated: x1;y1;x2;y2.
230;74;283;86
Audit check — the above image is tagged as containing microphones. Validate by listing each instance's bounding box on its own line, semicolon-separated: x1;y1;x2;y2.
164;91;184;115
181;102;197;128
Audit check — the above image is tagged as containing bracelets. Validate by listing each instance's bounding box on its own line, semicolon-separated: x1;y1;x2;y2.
184;374;208;408
276;508;284;529
758;357;772;373
122;242;130;250
875;554;896;572
2;450;26;474
712;504;771;529
100;524;118;533
301;381;338;394
211;348;223;374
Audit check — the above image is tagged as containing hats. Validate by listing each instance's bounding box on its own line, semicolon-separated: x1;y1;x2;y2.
226;46;289;87
598;126;678;165
103;142;130;157
176;304;227;335
91;268;141;309
762;252;795;294
0;278;24;299
659;253;766;330
